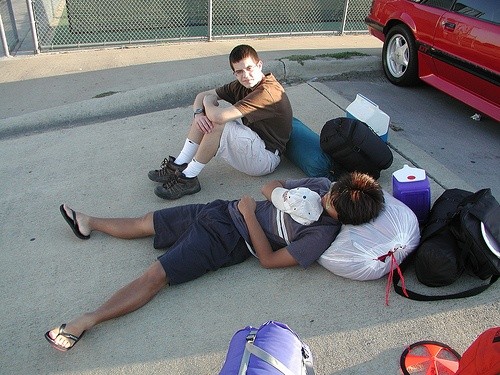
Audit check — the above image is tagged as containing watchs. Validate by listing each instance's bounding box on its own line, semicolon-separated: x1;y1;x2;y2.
193;108;205;117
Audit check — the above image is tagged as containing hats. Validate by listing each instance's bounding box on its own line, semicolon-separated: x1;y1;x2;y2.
270;186;323;227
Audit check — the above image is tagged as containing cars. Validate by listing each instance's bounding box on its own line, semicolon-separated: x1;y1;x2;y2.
363;1;500;123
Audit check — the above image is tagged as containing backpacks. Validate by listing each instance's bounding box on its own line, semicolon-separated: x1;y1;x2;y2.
319;117;393;181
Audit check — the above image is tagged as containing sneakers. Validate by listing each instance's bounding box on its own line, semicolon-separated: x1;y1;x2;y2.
154;168;202;201
148;155;189;183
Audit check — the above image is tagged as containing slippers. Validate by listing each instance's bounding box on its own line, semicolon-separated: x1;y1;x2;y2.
59;203;91;240
44;323;86;352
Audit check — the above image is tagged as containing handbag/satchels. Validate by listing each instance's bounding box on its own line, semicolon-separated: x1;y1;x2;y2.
392;187;500;301
217;321;317;374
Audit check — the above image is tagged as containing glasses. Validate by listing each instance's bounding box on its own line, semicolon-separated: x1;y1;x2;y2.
233;65;256;77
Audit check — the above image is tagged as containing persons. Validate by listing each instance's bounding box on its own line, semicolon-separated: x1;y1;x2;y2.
44;169;386;351
147;43;293;201
